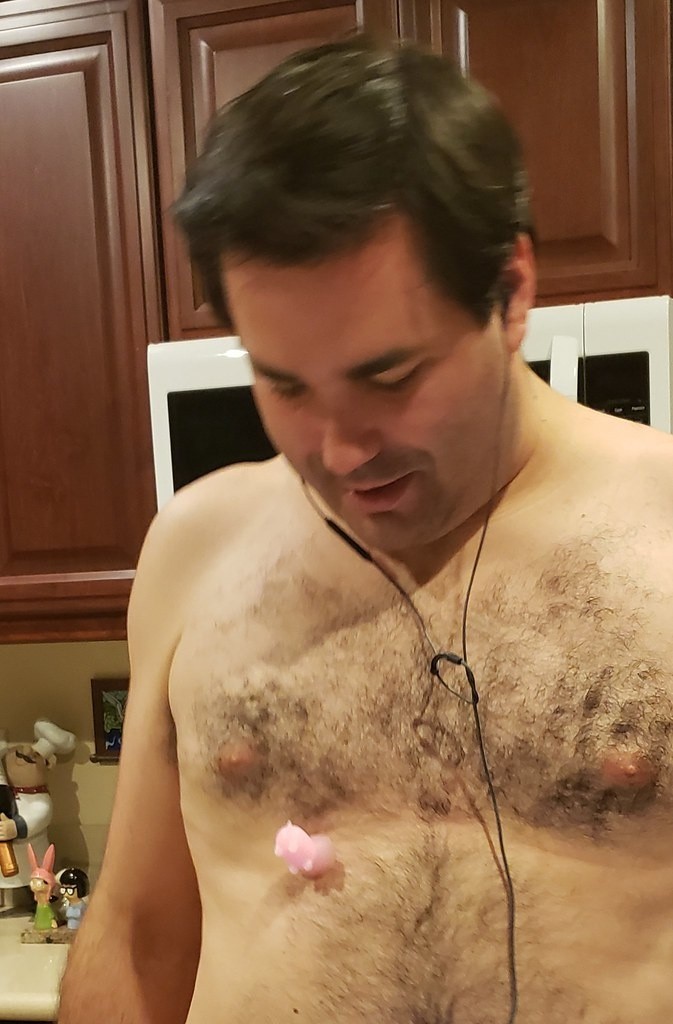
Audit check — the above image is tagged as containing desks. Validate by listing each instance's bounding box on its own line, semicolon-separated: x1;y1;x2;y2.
0;894;92;1021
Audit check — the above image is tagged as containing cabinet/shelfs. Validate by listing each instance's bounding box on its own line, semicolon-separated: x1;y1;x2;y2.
0;0;673;643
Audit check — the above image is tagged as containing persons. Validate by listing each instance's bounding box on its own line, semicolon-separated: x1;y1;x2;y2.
0;716;78;889
59;867;90;927
27;843;55;929
56;33;673;1024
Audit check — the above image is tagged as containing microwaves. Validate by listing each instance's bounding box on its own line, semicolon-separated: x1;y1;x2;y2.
142;296;673;578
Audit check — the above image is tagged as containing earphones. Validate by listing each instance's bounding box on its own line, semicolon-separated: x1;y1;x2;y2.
499;283;515;317
